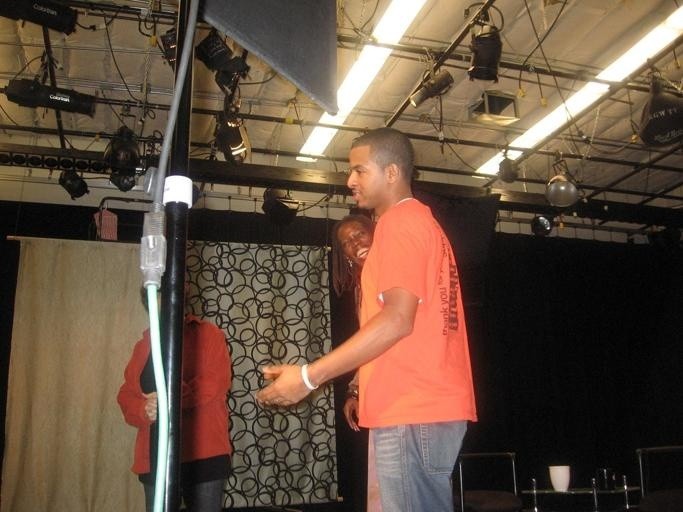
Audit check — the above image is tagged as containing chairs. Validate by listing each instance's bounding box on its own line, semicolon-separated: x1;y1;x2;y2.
449;443;681;511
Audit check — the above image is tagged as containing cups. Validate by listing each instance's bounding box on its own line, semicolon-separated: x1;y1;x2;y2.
595;464;619;493
548;465;571;495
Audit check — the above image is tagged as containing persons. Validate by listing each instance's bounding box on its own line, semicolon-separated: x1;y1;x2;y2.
116;276;231;511
253;127;480;511
332;214;383;509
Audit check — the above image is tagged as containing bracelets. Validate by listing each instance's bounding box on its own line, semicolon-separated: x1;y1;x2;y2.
344;385;359;401
301;363;320;390
